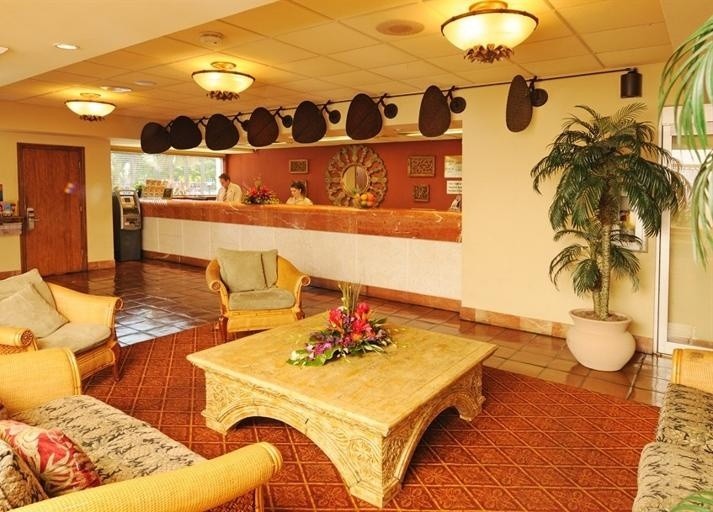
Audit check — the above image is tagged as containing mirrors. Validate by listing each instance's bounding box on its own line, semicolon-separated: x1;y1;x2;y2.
324;145;387;209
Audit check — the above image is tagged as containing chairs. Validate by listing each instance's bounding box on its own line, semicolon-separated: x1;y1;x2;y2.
208;248;310;342
1;271;123;384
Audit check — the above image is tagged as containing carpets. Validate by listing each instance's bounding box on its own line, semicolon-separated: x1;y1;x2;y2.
82;324;661;511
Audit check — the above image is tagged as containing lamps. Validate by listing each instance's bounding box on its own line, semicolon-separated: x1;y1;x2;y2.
192;63;255;99
440;0;539;61
66;94;116;122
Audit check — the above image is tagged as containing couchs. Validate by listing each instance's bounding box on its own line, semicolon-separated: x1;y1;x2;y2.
632;346;713;511
1;349;283;512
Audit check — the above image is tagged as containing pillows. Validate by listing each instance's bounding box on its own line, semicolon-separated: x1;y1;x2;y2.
0;281;70;340
263;248;278;286
1;420;101;497
217;247;267;292
1;440;49;512
0;267;57;309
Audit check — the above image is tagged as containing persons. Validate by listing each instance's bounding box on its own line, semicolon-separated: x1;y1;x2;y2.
285;182;313;205
215;174;242;202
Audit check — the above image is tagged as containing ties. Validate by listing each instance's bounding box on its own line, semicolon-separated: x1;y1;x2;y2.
223;188;227;201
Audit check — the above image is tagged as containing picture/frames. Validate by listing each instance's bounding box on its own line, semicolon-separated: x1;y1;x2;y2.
407;156;436;177
289;159;308;173
610;180;649;253
412;184;429;202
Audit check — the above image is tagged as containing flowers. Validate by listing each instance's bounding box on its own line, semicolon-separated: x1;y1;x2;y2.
241;184;279;204
285;280;404;362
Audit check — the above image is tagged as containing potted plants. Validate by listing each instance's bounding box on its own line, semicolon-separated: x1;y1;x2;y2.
531;103;694;370
